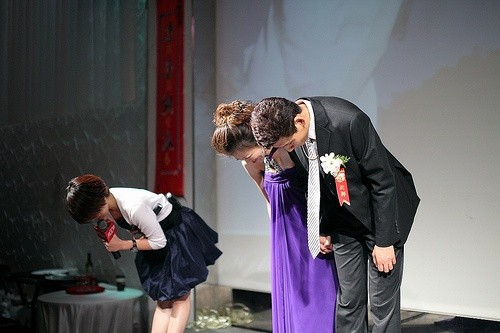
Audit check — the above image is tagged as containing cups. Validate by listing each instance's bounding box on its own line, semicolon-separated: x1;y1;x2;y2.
115;275;126;291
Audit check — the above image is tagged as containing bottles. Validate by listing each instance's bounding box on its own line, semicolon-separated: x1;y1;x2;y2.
84;253;94;275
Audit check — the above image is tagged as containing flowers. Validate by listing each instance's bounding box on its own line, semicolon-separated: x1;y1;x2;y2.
320;152;350;176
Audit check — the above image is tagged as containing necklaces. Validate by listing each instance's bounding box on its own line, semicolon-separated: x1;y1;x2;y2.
302;145;319;160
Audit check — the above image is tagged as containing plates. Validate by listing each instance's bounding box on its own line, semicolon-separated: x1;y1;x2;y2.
66;286;105;293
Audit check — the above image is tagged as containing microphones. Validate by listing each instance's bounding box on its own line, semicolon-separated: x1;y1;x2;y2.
93;217;122;260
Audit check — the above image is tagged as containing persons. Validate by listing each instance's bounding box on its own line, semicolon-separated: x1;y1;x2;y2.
212;99;340;333
65;173;222;333
250;96;420;333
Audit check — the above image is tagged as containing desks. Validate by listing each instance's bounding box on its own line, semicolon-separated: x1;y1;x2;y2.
38;283;142;333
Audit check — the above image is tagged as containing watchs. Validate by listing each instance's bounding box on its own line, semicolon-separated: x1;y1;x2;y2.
129;239;139;254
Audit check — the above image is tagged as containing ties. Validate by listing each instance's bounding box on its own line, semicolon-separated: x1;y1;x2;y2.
305;137;320;259
